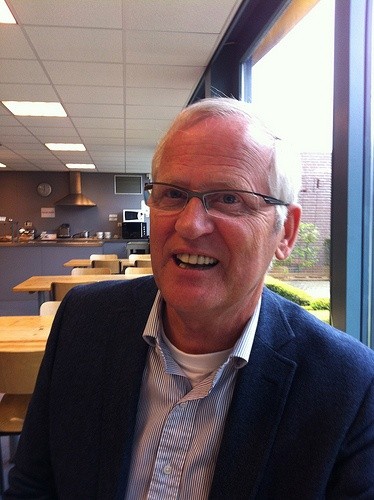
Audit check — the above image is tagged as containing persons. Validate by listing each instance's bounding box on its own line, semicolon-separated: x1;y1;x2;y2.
4;95;374;500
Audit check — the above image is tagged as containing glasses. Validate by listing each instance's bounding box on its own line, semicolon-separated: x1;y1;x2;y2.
143;182;290;221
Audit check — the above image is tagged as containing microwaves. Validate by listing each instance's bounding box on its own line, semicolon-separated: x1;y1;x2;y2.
122;208;144;222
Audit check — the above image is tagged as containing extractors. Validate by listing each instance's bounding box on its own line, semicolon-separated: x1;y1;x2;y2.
55;171;96;207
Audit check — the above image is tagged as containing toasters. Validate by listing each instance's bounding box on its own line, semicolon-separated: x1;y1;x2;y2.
57;224;71;238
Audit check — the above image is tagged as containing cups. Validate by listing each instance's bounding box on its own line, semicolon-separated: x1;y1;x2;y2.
96;232;103;239
104;232;111;239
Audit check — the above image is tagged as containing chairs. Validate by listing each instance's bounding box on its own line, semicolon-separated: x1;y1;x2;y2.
0;255;152;436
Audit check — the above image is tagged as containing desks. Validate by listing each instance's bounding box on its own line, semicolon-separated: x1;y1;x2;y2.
0;316;56;351
12;274;149;307
64;259;134;267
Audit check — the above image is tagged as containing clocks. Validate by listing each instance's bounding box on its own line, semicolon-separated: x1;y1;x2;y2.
37;182;52;197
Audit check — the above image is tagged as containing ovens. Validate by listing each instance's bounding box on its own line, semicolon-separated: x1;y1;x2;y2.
122;222;147;239
